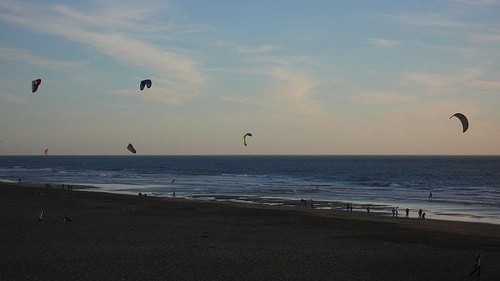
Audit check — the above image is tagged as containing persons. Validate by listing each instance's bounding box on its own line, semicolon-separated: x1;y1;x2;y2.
144;194;149;198
405;208;409;218
396;206;399;217
466;253;484;279
367;206;370;215
300;198;304;207
427;191;433;200
422;212;425;219
170;178;175;184
38;209;45;221
350;206;353;211
392;207;396;218
173;191;176;198
346;202;350;212
63;214;72;223
42;181;76;193
304;199;307;206
310;199;313;208
138;193;143;198
418;209;422;219
